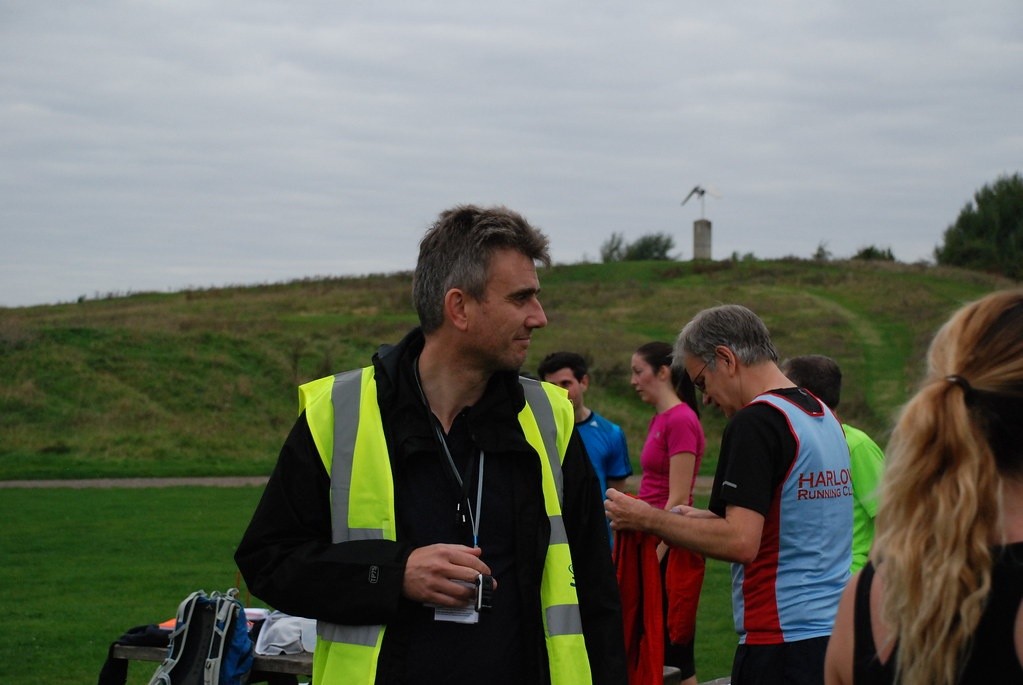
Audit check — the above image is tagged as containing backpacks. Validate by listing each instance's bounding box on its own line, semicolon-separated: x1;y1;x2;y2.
147;587;255;685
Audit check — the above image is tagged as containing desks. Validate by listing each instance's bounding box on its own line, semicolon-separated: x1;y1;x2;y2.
113;644;314;685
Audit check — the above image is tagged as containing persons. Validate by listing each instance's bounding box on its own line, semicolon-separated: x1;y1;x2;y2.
630;343;707;685
538;351;633;554
780;355;884;577
824;289;1023;685
605;305;853;685
235;205;629;685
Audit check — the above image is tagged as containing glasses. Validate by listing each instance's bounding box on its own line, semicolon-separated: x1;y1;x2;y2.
690;344;734;394
